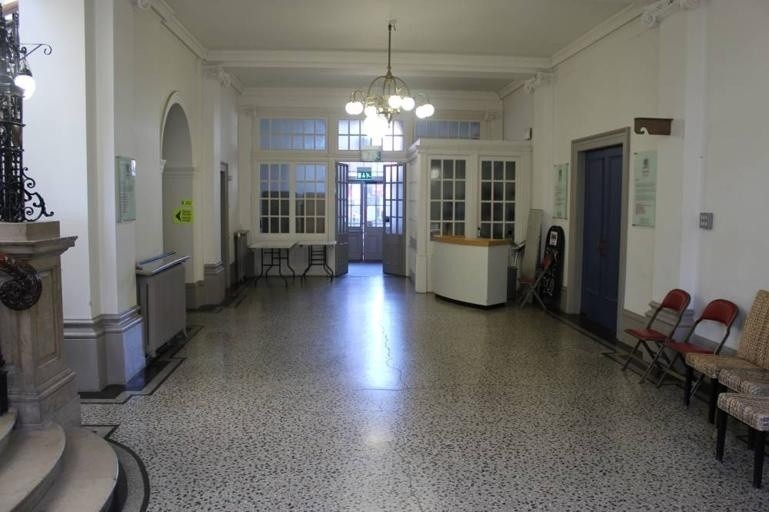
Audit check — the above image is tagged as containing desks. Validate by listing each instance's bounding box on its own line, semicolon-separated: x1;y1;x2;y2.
247;239;337;287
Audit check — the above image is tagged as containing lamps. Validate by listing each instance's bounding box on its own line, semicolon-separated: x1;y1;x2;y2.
346;20;435;140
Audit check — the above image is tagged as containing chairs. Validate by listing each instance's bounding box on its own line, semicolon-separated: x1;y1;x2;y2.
621;288;769;492
518;255;551;311
